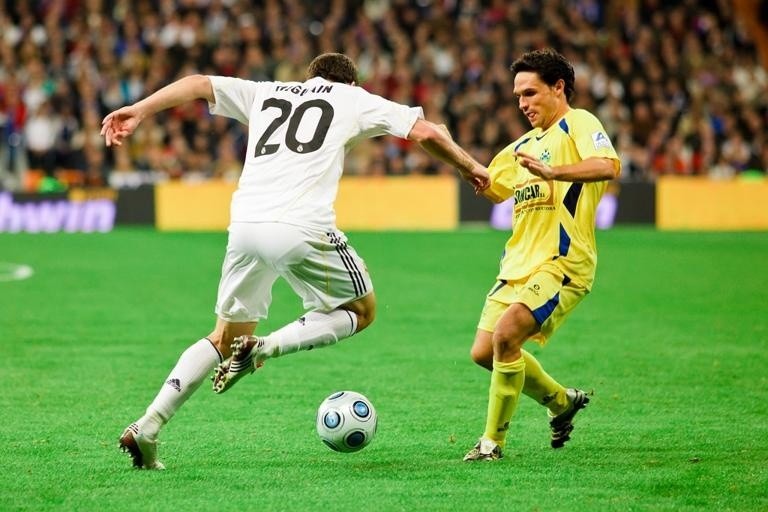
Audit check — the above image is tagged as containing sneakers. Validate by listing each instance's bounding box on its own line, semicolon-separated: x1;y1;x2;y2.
547;389;590;449
210;335;265;393
463;438;504;462
119;423;165;470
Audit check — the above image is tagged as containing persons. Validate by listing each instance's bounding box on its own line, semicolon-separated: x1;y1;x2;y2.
436;47;621;463
1;1;768;193
100;52;490;470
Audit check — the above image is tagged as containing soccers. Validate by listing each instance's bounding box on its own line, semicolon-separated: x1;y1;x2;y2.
316;391;378;453
